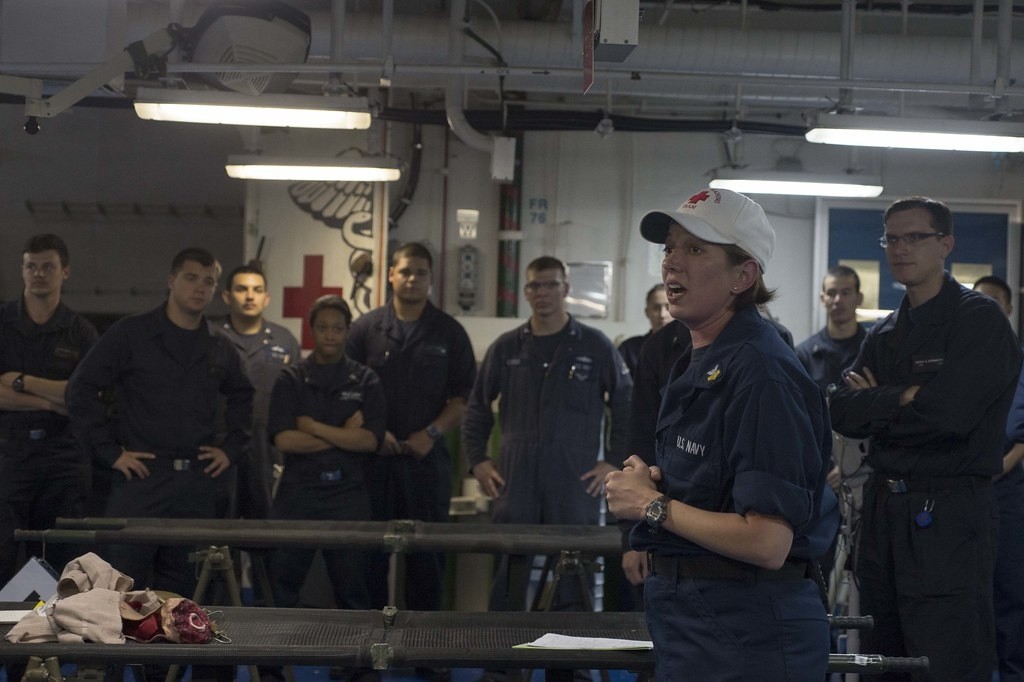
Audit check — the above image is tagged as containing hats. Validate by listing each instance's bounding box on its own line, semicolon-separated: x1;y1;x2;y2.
639;188;776;273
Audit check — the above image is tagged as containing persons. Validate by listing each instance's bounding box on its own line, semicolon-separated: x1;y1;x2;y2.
796;196;1024;682
259;243;478;682
462;254;634;682
580;189;844;682
65;248;302;682
0;234;101;682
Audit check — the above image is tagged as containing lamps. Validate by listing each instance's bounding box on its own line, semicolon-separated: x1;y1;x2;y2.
707;106;1024;199
133;86;402;184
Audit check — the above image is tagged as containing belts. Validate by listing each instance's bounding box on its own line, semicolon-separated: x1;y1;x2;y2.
877;477;987;492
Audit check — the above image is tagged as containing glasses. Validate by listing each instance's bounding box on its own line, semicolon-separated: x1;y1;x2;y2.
879;230;943;248
524;280;564;290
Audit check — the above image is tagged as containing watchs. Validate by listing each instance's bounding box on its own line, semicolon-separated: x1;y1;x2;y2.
646;494;672;528
13;373;26;392
426;423;441;440
